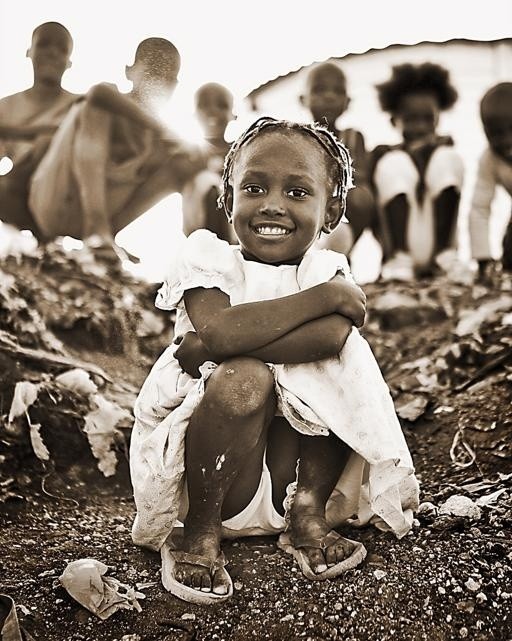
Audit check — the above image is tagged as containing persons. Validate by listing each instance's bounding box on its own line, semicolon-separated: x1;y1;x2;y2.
27;38;209;267
297;65;374;241
1;21;85;243
180;83;239;246
127;114;420;606
467;80;511;284
367;62;465;286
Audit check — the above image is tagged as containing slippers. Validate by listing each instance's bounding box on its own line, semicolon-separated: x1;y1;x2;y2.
160;529;234;607
84;237;122;275
277;529;367;582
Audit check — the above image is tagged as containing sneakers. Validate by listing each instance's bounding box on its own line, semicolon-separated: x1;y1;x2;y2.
431;247;477;279
380;251;417;285
